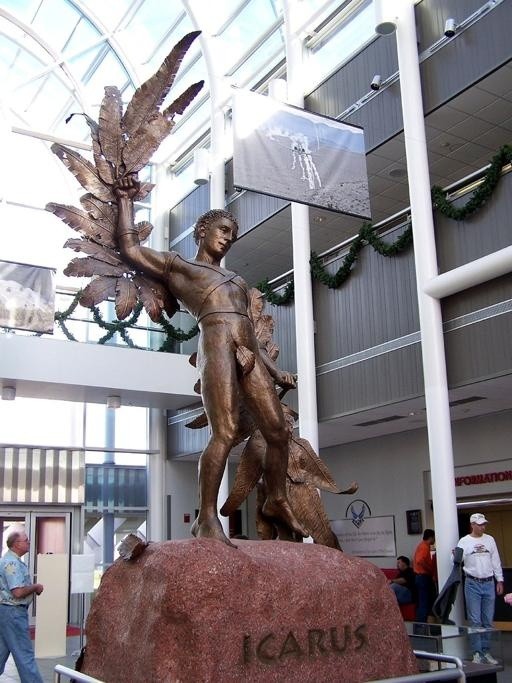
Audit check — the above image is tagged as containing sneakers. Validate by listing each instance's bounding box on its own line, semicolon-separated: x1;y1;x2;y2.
485;652;499;665
472;652;484;665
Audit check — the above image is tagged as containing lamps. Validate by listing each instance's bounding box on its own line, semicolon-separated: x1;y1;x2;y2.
443;17;460;37
1;385;16;400
106;393;121;409
369;73;383;90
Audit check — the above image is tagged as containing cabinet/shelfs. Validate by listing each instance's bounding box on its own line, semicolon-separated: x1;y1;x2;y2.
402;620;505;682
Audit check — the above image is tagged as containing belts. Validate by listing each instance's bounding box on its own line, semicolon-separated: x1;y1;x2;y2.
467;574;493;582
15;604;29;609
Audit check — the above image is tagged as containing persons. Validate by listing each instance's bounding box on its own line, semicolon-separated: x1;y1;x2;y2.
449;512;506;666
108;174;313;554
385;555;414;605
0;529;48;683
411;528;436;622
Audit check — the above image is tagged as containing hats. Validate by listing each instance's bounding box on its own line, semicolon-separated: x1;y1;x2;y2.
470;513;489;525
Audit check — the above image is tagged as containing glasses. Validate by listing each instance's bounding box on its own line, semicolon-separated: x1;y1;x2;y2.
14;538;31;544
